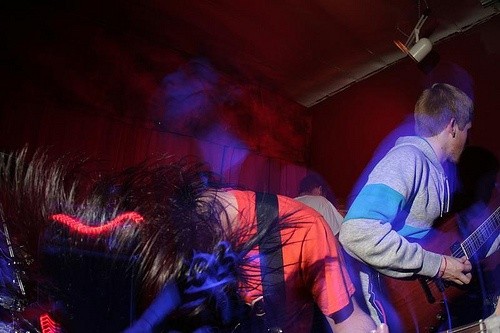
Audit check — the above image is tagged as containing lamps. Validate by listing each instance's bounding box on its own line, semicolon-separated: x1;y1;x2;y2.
393;8;439;63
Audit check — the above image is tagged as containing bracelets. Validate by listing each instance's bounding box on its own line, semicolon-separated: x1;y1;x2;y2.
438;255;447;279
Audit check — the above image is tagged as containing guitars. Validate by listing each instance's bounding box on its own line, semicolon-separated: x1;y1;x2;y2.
372;206;500;333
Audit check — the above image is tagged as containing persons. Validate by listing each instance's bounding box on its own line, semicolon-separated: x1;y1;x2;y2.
290;174;345;241
339;81;474;333
0;143;387;333
